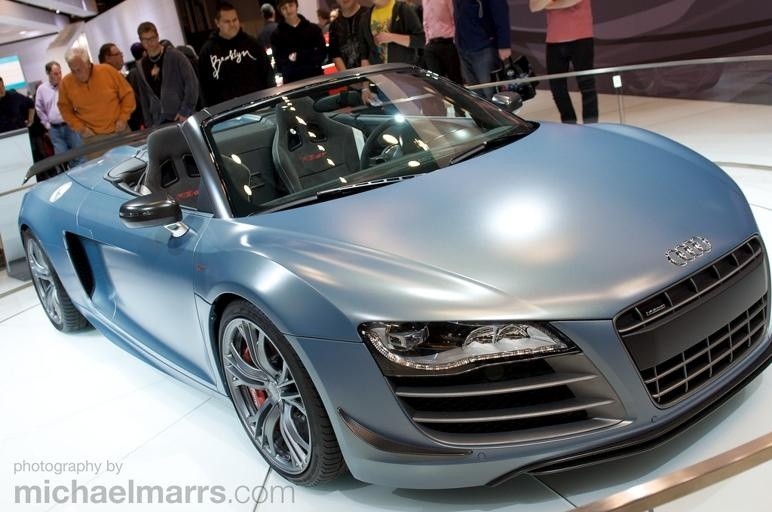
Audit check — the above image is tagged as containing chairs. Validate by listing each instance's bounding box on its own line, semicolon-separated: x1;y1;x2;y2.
130;121;258;224
267;95;366;197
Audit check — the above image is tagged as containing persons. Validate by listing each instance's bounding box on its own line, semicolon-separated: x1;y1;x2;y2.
529;0;599;125
0;0;512;184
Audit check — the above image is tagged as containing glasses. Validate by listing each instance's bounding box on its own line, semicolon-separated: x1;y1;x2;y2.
140;36;159;43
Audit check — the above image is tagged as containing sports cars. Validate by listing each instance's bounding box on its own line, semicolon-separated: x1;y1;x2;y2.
18;62;772;490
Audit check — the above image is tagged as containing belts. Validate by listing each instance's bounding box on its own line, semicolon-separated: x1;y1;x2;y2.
49;122;67;129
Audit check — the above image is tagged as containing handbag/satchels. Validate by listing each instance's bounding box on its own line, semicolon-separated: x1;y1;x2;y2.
490;54;541;101
